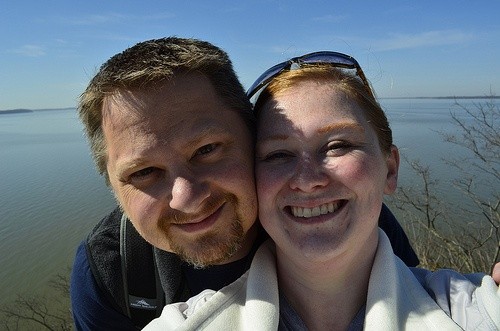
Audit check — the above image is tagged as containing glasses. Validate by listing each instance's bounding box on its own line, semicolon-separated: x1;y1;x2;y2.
245;51;375;109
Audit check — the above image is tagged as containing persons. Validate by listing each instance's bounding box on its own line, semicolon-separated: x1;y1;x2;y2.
139;65;500;331
72;37;419;331
